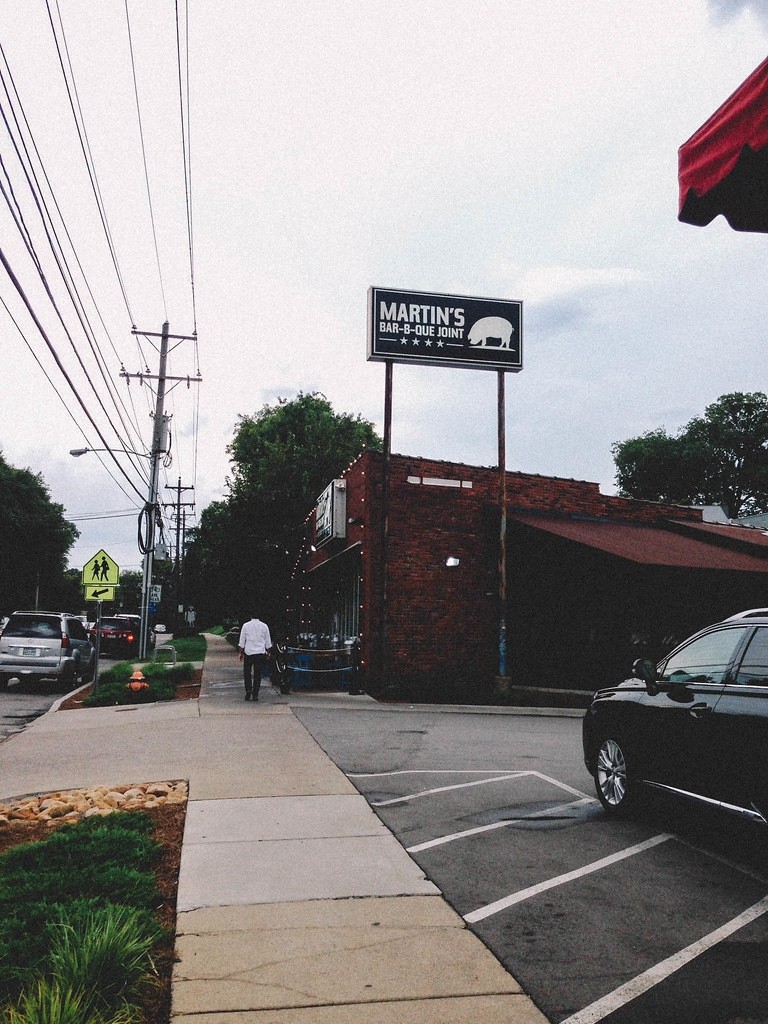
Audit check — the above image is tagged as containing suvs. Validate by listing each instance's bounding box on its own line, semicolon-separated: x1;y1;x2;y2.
0;611;97;696
0;613;158;660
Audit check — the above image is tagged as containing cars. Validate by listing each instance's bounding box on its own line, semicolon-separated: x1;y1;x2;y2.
153;623;169;635
580;606;767;884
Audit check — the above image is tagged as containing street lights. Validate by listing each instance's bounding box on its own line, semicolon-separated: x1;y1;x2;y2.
69;448;161;662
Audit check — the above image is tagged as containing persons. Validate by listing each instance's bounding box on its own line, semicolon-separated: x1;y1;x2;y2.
238;610;272;700
2;616;10;629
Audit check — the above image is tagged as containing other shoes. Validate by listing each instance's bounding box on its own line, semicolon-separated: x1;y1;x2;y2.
253;696;258;701
245;690;251;702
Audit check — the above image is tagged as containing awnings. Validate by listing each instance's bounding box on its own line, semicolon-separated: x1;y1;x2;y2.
508;507;768;572
678;56;768;234
